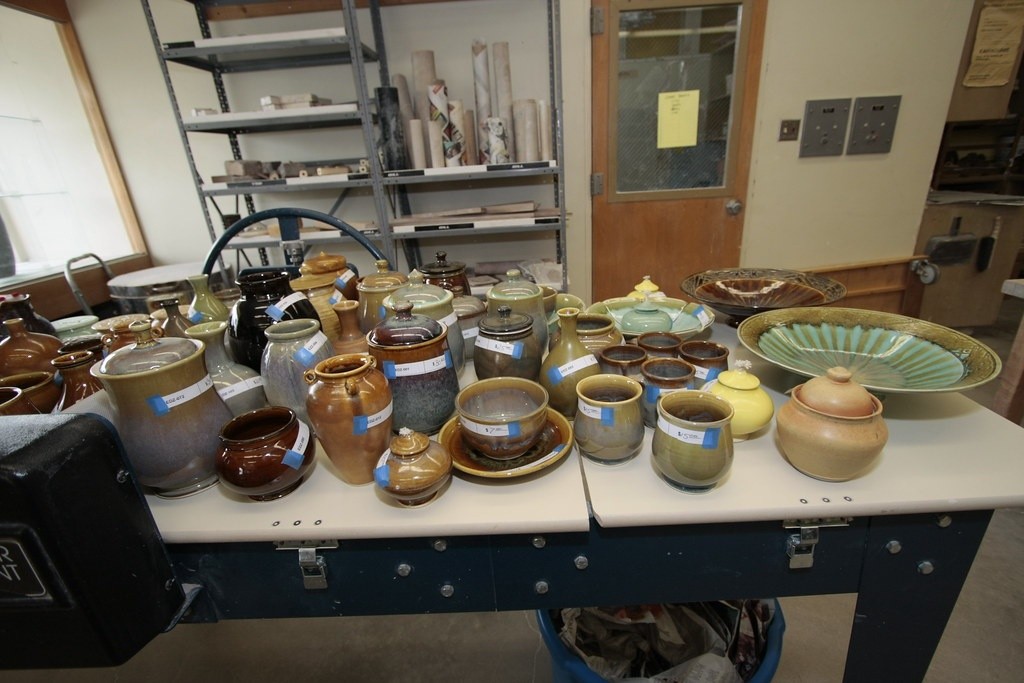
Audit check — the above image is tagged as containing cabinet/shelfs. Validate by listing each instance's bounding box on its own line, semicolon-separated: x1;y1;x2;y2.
141;0;568;301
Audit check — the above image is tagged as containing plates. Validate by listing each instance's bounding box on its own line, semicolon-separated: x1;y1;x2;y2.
587;296;715;342
438;406;573;478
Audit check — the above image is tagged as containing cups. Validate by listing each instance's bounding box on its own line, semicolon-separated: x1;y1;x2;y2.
573;375;646;464
542;286;557;313
0;387;33;414
638;331;683;358
637;357;696;431
0;371;62;414
680;339;730;390
597;345;648;381
652;391;735;494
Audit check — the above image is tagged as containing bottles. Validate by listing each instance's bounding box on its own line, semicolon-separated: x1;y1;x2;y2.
539;307;601;422
185;321;266;418
331;300;370;355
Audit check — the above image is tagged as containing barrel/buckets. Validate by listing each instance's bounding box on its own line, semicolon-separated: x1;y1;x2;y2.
537;597;786;683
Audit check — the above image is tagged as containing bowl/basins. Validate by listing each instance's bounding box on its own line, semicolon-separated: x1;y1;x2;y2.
736;307;1002;404
455;377;549;459
680;267;848;328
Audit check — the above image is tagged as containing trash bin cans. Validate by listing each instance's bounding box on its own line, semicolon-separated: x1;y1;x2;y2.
535;597;785;683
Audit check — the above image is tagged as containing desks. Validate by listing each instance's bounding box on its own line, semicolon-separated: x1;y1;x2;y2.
990;279;1024;427
141;321;1024;683
